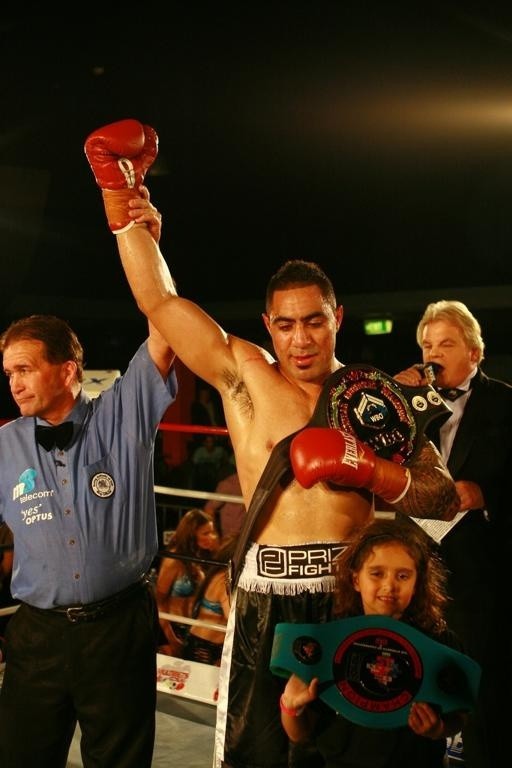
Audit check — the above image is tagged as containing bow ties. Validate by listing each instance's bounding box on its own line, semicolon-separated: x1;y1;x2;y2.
437;387;468;402
35;421;75;452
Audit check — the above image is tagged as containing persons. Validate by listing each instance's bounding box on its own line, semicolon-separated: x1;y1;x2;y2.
391;299;512;768
279;518;471;768
155;386;247;668
0;184;179;768
84;119;461;768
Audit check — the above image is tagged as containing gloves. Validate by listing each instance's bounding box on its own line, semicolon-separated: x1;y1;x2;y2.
289;425;412;504
81;117;159;235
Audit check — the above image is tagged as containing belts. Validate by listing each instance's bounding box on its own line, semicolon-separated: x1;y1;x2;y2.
20;571;151;625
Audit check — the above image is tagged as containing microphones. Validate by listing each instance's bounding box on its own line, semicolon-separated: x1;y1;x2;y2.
417;361;446;379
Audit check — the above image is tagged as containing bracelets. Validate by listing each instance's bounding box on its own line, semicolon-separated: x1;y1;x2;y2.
279;693;305;718
